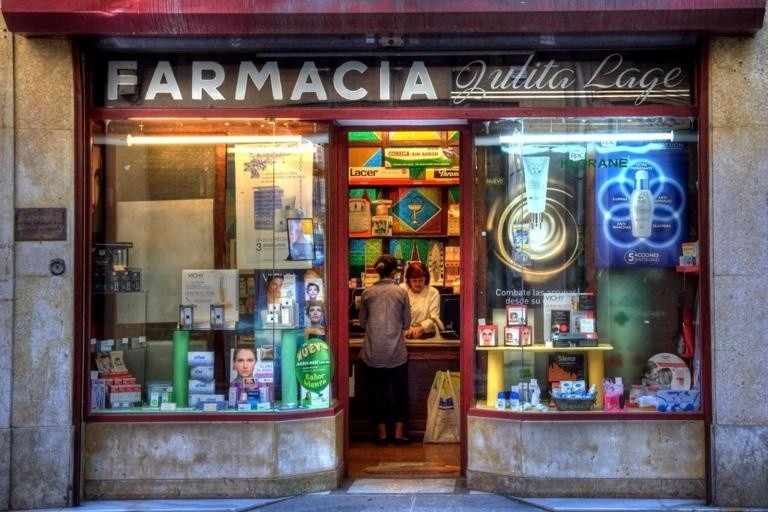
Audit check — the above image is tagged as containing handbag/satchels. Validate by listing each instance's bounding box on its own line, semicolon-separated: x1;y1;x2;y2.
423;369;462;445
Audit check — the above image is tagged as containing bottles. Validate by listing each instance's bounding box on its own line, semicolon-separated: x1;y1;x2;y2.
496;376;624;413
226;380;272;411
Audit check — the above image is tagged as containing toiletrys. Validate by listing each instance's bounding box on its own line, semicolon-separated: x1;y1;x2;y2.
522;155;550;230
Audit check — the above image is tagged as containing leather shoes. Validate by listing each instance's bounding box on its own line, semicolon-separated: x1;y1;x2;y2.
367;436;388;446
394;437;413;446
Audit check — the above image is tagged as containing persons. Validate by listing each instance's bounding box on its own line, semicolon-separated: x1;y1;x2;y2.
644;361;673;391
230;345;258;382
305;302;326;329
306;282;320;300
398;262;441;339
359;254;413;445
244;378;255;388
480;312;530;347
604;378;615;395
265;274;285;304
